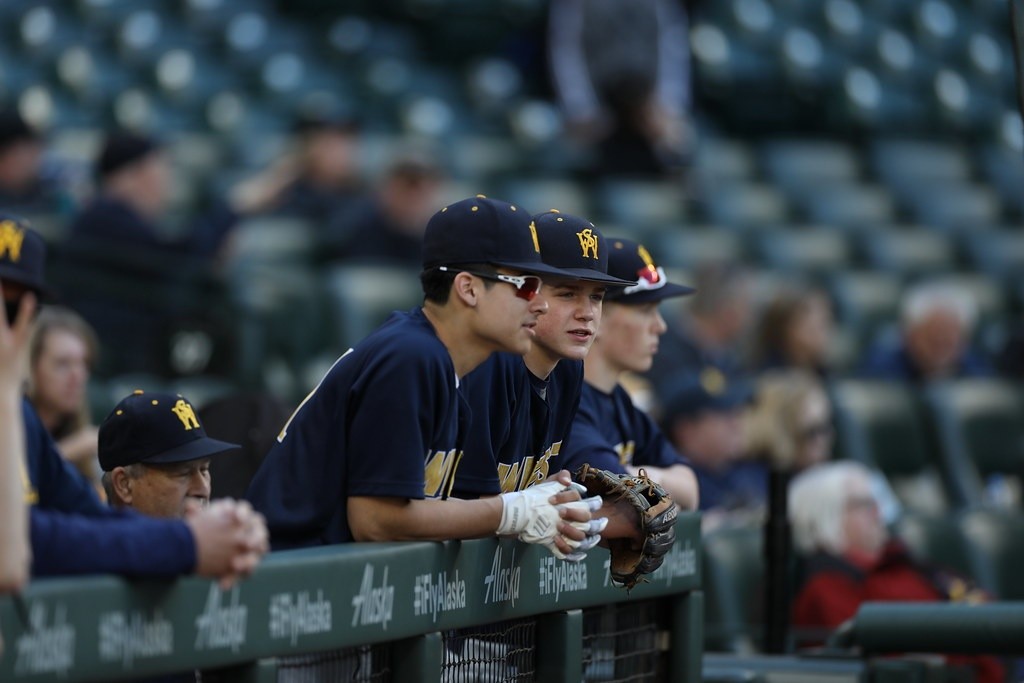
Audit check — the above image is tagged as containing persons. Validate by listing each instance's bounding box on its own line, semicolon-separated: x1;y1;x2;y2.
0;213;60;597
244;191;579;683
851;274;996;384
755;288;846;379
21;302;106;504
441;209;681;683
782;458;1013;683
752;364;833;490
96;386;252;682
546;0;700;184
560;234;702;683
0;99;452;379
652;258;797;518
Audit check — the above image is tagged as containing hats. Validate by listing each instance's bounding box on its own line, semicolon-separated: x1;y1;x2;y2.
0;207;46;291
599;237;698;306
529;208;639;288
97;389;243;473
423;194;581;284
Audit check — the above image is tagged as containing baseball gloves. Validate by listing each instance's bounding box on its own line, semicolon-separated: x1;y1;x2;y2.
575;464;679;593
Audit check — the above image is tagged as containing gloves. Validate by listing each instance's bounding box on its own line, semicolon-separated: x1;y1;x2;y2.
494;481;609;564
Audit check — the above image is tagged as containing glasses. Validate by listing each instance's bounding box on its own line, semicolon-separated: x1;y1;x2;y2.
434;265;543;302
